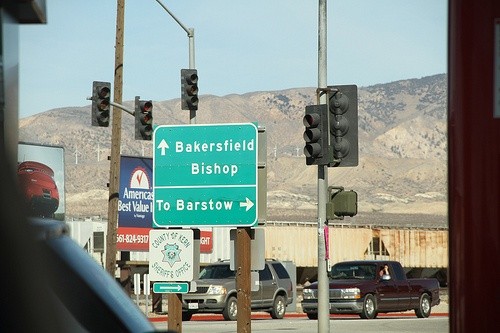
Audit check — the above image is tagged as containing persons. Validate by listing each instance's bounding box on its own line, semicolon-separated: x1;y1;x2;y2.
378;264;389;276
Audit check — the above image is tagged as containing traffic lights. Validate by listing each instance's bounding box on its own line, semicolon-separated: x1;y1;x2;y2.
181;68;199;111
92;81;111;127
303;105;332;166
133;99;153;141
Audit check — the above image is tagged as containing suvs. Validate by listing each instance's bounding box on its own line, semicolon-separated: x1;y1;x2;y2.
180;259;295;323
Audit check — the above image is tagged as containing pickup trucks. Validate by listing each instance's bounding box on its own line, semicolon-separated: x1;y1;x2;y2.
301;260;441;320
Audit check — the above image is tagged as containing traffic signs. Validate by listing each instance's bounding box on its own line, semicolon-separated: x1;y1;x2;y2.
153;123;259;228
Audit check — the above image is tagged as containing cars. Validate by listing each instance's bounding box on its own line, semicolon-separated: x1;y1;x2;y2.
15;161;59;216
